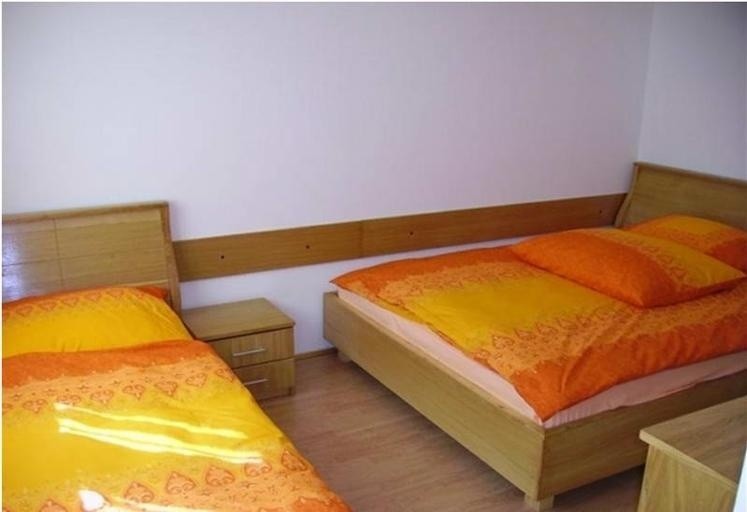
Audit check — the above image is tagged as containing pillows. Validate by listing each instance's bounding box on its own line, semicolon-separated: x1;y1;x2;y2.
627;212;747;272
506;226;747;310
1;285;191;362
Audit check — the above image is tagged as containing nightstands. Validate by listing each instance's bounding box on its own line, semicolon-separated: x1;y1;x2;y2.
181;297;297;402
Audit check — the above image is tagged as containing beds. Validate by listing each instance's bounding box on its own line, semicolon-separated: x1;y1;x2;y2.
2;203;354;512
322;162;747;512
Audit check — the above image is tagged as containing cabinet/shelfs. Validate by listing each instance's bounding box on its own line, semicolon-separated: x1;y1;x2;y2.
636;396;747;512
